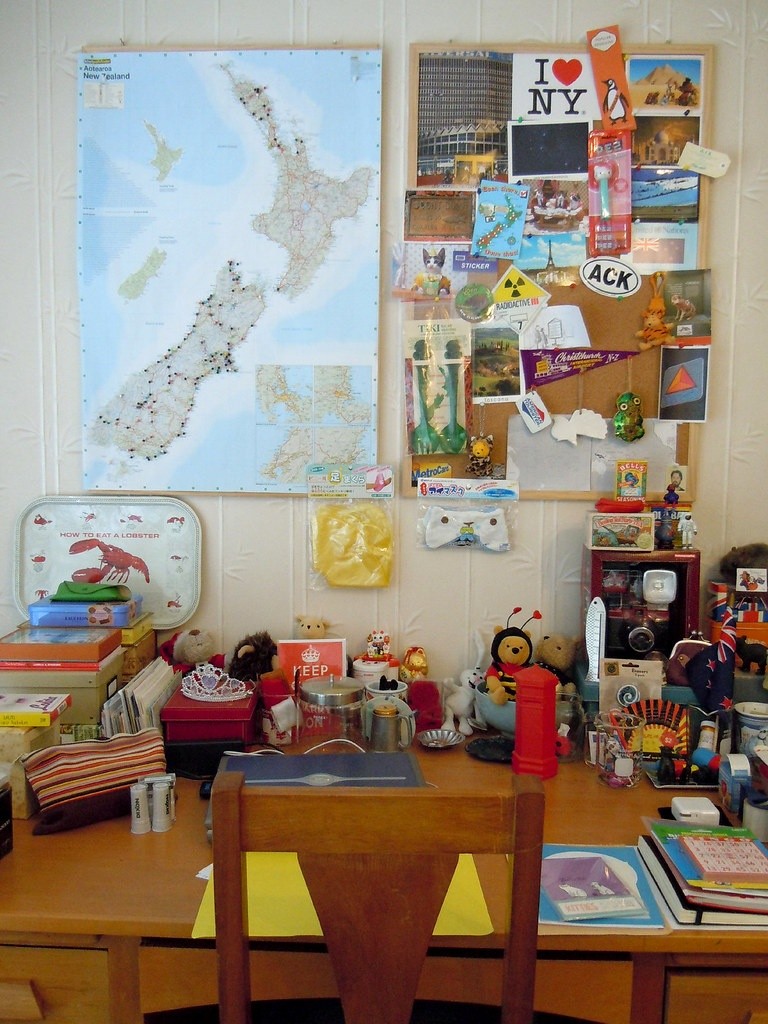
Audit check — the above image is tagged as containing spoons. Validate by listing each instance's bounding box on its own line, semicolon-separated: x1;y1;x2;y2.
439;340;467;453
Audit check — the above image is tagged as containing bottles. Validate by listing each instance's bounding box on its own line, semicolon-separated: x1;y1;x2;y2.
298;670;367;760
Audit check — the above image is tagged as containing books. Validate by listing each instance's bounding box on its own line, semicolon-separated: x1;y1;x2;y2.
538;820;768;936
0;645;121;671
102;656;183;738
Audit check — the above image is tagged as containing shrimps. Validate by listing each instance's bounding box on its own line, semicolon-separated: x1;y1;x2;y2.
35;590;50;599
34;514;53;524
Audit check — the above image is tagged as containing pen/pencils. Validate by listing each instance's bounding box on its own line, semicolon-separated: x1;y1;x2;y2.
293;667;302;744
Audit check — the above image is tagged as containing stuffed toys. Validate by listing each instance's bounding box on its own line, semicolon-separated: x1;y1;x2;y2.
295;614;330;639
465;435;494;476
441;607;583;763
633;306;676;350
159;629;227;681
227;631;279;682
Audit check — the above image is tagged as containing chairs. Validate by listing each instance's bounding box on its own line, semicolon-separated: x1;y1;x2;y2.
210;770;544;1024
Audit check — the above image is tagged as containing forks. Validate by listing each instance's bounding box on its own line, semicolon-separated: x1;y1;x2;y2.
411;339;439;456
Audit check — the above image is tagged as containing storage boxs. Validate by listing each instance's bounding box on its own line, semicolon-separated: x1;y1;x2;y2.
584;510;655;552
700;617;768;646
160;681;259;742
614;458;648;503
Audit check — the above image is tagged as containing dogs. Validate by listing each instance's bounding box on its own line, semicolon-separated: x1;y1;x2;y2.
670;295;697;321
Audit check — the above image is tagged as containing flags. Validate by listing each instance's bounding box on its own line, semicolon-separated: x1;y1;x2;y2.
684;607;736;716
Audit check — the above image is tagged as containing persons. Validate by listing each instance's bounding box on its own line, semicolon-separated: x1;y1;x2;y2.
671;470;685;491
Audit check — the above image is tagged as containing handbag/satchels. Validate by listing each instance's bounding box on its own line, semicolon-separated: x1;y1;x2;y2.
14;726;177;835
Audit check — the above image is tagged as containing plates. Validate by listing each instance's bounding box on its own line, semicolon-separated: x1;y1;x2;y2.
360;697;416;745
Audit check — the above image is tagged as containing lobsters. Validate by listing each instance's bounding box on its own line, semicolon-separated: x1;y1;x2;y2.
70;538;150;586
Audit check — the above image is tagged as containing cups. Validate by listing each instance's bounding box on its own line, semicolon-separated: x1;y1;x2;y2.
597;712;645;788
369;705;412;754
732;701;768;754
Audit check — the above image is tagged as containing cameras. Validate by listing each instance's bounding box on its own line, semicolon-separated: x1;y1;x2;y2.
605;601;667;658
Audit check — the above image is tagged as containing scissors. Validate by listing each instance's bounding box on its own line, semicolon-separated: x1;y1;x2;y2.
599;710;627;749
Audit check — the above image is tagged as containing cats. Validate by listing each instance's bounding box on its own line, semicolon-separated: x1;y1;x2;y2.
419;249;446;272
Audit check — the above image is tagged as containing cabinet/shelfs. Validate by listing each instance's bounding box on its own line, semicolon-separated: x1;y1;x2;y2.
0;722;768;1024
